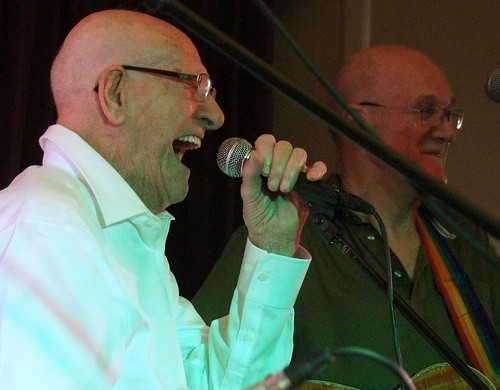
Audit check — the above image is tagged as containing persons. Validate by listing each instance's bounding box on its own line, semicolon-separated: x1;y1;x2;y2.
0;9;328;390
191;44;500;390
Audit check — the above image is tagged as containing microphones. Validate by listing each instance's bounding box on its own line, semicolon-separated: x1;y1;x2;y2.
486;69;500;103
246;347;336;390
216;137;377;216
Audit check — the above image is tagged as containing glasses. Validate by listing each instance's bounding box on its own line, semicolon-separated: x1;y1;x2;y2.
359;102;465;130
94;65;217;101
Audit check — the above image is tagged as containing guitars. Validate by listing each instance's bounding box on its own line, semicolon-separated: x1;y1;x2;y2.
297;362;498;390
